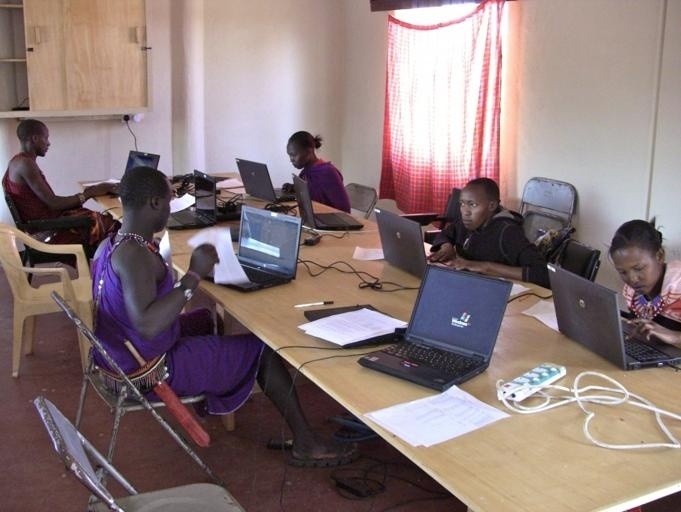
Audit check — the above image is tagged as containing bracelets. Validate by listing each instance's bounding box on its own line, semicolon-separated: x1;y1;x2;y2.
174;281;193;301
76;193;85;203
187;269;201;281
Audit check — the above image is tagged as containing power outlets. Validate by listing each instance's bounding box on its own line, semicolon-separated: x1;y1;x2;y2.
121;115;131;124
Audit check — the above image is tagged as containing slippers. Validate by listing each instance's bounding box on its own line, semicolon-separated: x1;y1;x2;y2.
288;439;364;467
332;422;380;442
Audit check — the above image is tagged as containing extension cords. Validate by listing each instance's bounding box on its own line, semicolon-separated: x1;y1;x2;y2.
497;361;567;403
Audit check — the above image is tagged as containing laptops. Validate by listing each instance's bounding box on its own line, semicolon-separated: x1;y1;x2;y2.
234;157;297;202
357;264;513;394
106;150;161;199
546;261;681;371
166;168;217;231
291;171;365;231
373;206;452;281
202;204;302;293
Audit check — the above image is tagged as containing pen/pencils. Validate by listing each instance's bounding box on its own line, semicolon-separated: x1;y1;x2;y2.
293;300;335;309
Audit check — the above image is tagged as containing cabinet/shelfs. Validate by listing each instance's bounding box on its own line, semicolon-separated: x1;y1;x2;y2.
0;0;153;119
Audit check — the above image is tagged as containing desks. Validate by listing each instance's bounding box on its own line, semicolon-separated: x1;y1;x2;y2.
80;170;681;512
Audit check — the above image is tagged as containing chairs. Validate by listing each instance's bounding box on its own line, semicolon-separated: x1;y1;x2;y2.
519;176;577;245
1;180;95;286
0;223;94;379
344;182;378;219
533;227;601;292
33;395;248;511
398;187;461;244
50;290;226;510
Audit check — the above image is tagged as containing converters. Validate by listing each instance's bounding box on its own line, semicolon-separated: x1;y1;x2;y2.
303;233;321;245
270;204;289;212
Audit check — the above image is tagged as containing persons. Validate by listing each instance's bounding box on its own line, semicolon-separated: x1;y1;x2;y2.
430;178;552;289
282;131;351;214
93;166;359;459
5;118;119;269
609;219;681;350
